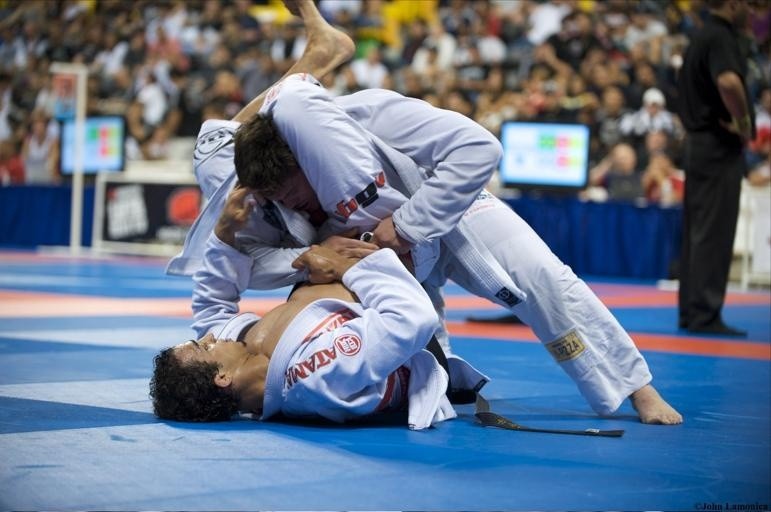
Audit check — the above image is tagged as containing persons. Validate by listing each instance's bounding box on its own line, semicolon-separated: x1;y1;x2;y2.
213;87;683;428
674;0;753;336
746;1;771;188
323;1;687;208
1;0;306;185
148;0;491;432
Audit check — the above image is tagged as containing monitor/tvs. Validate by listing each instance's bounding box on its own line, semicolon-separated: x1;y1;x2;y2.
499;121;592;190
58;115;128;177
